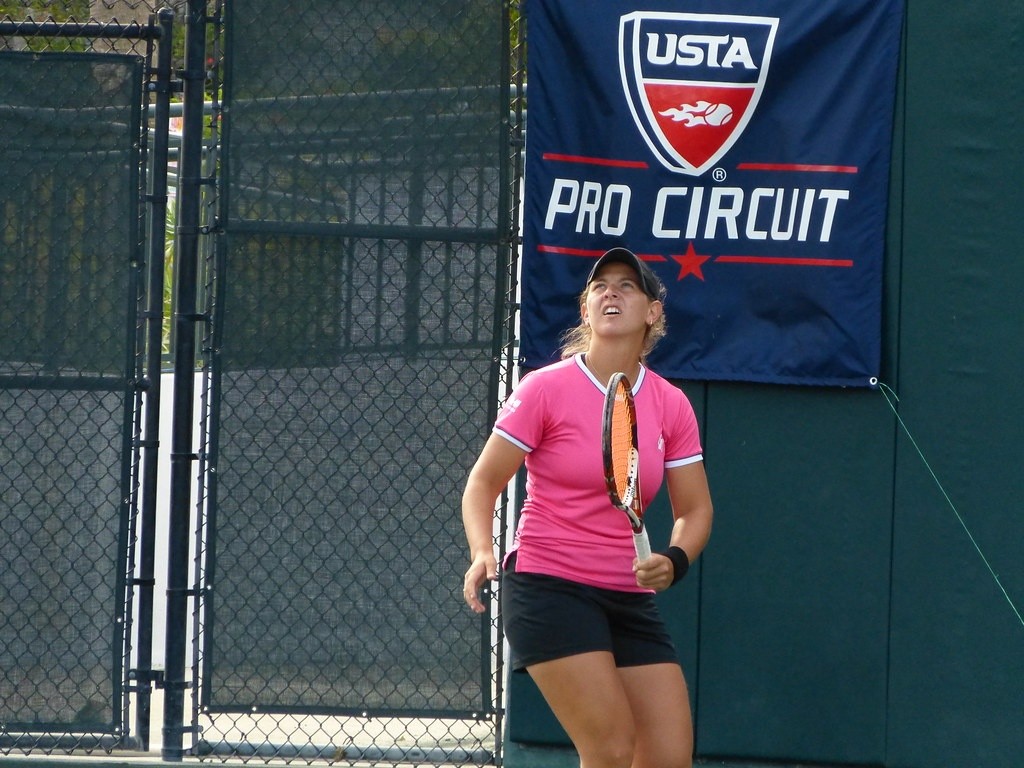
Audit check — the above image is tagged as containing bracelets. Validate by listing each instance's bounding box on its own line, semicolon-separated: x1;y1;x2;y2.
662;545;690;585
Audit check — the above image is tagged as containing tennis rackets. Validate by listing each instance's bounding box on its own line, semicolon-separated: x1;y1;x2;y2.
600;372;654;562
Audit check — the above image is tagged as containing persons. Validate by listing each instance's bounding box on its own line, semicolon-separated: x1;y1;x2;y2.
462;245;714;768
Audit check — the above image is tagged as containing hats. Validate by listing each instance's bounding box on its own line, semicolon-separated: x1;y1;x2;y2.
587;248;658;299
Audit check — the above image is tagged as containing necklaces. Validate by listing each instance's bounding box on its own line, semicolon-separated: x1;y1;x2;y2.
587;354;609;388
